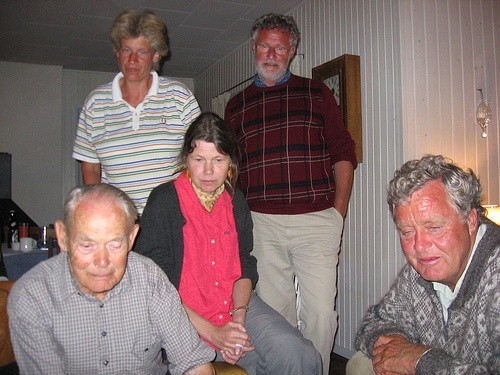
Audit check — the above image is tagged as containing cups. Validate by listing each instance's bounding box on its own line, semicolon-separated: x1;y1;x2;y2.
41;226;48;244
11;242;20;251
19;237;37;253
19;223;29;238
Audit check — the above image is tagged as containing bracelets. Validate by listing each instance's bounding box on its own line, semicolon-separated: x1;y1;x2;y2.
230;303;249;316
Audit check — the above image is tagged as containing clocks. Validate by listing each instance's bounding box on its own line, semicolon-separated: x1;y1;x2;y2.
312;54;363;163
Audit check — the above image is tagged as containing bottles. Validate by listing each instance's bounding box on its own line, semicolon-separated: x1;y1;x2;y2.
8;210;18;248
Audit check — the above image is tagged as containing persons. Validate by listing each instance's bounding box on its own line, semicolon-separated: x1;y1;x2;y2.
135;111;323;375
73;5;202;252
7;183;212;375
345;155;500;375
224;13;358;375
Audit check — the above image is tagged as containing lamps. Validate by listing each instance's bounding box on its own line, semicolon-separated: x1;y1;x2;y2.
480;205;500;226
